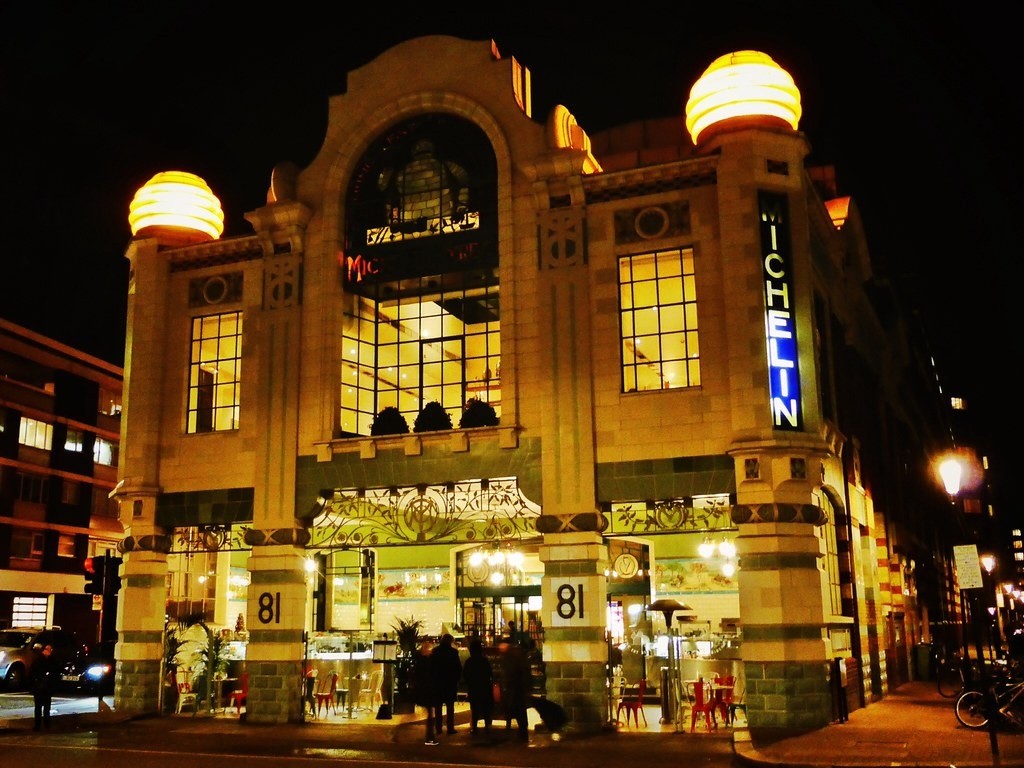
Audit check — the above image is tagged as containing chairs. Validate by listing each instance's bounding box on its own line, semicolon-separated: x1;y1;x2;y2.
164;667;384;720
607;671;746;732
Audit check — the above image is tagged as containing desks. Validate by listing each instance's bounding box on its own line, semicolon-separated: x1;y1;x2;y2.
210;677;238;714
702;685;733;728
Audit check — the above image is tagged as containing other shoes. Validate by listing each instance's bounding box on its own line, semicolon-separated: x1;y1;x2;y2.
484;727;490;734
32;726;40;732
447;729;458;734
513;735;527;741
436;728;442;734
470;730;478;736
424;737;439;745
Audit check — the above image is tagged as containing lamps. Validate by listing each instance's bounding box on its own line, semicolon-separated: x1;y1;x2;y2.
698;497;737;579
684;48;804;148
469;503;528;585
128;170;227;246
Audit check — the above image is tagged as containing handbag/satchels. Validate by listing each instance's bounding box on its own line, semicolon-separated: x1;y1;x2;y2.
375;700;393;720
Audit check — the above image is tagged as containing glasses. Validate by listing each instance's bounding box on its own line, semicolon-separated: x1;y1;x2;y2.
45;648;53;651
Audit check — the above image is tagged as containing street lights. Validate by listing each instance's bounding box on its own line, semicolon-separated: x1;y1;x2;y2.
936;455;970;707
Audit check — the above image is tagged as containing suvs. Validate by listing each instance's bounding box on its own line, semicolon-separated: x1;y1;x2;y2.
0;624;74;692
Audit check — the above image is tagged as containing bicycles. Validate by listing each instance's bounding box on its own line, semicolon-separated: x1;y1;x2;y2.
933;649;1024;731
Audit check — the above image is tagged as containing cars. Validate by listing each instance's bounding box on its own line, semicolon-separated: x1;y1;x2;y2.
42;639;118;698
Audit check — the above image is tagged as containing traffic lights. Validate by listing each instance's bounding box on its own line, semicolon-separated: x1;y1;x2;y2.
83;554;104;595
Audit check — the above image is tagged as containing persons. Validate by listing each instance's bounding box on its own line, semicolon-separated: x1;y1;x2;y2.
30;642;59;730
414;620;532;746
